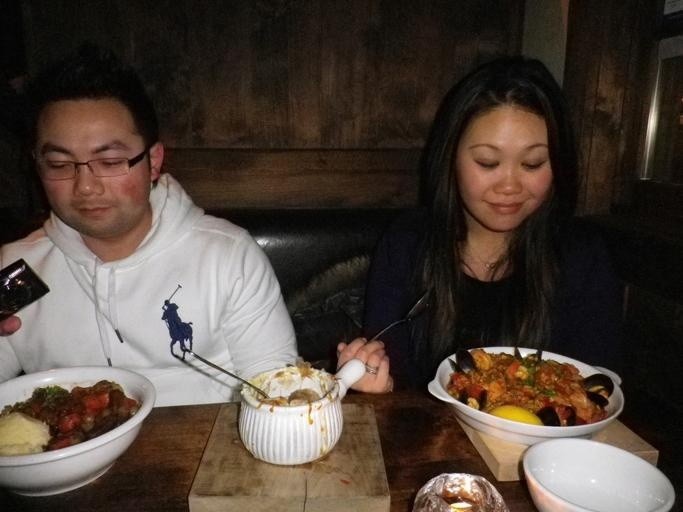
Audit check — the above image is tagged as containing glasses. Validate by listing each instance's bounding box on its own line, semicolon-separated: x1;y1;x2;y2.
28;145;153;181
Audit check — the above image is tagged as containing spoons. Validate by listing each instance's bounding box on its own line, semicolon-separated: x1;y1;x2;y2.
367;286;435;347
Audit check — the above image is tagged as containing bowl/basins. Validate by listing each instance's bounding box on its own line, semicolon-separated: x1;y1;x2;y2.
524;438;677;512
426;345;627;446
0;366;156;496
236;357;365;468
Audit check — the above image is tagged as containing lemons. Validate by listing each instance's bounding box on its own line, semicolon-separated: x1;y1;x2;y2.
488;404;543;426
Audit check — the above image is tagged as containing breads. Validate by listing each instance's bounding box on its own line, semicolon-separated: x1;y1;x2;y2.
412;473;509;512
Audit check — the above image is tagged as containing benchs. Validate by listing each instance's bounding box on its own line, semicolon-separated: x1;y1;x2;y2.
201;209;419;372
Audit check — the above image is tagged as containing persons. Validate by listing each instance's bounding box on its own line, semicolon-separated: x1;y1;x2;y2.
0;45;298;412
338;49;625;399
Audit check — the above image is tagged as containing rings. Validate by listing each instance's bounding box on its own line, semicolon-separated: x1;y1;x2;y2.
364;363;380;375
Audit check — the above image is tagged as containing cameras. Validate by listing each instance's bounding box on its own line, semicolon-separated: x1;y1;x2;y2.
0;259;49;321
